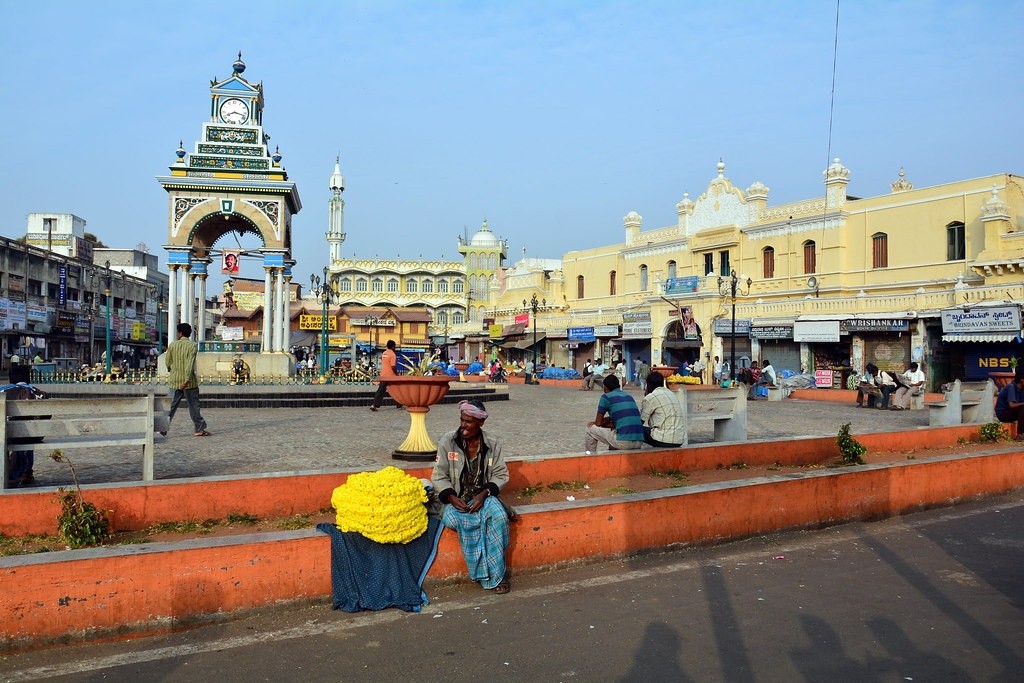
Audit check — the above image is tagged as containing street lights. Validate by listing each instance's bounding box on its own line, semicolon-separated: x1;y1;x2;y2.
523;293;546;373
90;260;126;384
718;269;752;381
365;313;379;368
310;266;340;385
150;280;170;357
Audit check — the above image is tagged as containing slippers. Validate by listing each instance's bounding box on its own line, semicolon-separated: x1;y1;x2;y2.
157;431;167;436
397;407;405;411
369;407;378;411
197;431;211;436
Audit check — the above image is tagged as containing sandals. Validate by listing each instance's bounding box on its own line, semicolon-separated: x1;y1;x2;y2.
494;580;510;594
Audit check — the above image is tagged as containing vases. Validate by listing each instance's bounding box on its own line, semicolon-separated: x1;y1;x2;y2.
453;364;470;371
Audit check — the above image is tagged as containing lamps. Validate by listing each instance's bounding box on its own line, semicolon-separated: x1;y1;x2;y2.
807;277;819;298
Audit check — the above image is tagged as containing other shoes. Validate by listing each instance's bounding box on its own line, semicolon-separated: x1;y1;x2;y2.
888;404;902;411
879;406;887;410
1015;436;1024;441
856;404;863;408
746;397;754;400
863;405;873;408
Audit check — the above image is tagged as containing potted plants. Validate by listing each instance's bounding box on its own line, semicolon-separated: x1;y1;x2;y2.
1008;354;1021;373
377;351;454;410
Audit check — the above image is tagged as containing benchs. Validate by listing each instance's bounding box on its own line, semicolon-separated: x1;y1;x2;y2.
594;368;622;391
759;374;784;401
874;379;928;410
922;378;994;426
0;383;173;490
679;382;748;446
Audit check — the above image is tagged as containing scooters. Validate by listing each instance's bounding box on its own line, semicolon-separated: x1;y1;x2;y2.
488;364;508;383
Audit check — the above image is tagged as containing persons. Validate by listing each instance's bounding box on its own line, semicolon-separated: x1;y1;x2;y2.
585;374;645;452
995;368;1024;441
11;353;20;364
856;362;925;410
296;355;316;375
445;357;454;369
460;356;464;362
223;254;238;271
489;358;535;384
579;358;627;391
474;356;478;362
369;340;404;411
693;357;706;384
640;372;685;448
156;323;211;436
432;399;518;594
334;357;373;377
34;351;44;363
612;345;622;368
713;356;776;399
632;357;650;390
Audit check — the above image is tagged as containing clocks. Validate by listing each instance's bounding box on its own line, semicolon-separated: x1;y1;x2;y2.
219;98;250;125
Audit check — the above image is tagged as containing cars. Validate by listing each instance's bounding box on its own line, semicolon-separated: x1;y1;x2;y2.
722;355;751;377
334;355;362;376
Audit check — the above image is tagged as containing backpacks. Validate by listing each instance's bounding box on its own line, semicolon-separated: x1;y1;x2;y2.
880;371;901;390
